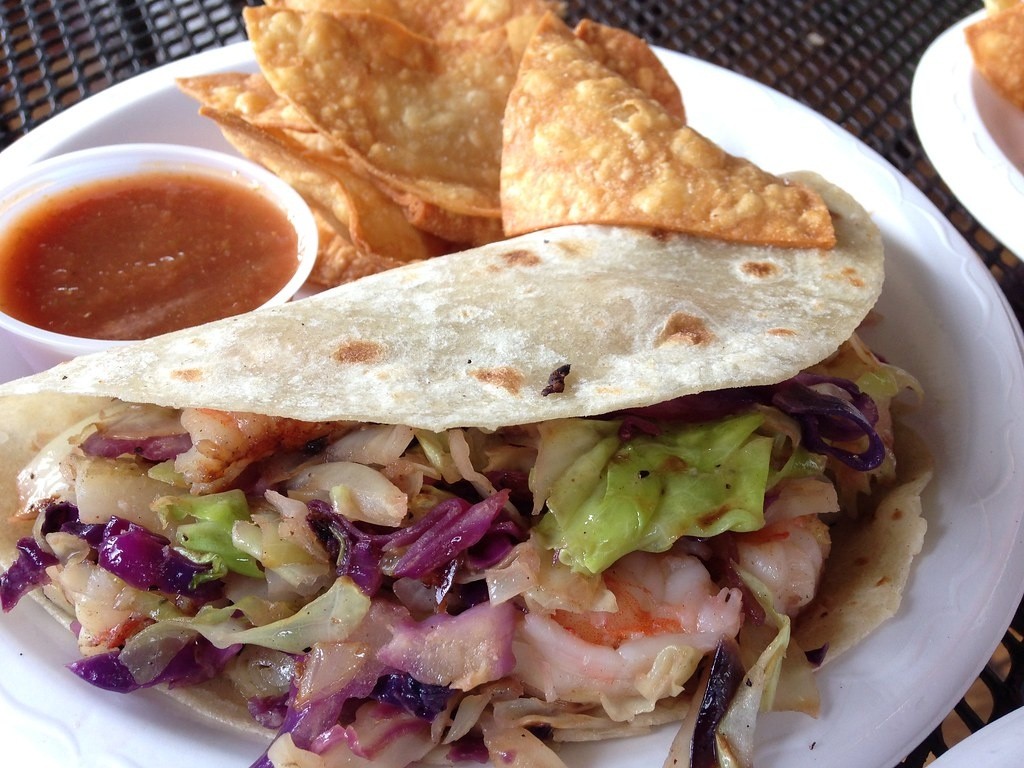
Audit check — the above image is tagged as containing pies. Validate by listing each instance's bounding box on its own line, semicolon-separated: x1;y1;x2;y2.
0;168;930;768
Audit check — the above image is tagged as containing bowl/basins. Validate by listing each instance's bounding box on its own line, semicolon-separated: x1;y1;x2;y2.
0;141;320;355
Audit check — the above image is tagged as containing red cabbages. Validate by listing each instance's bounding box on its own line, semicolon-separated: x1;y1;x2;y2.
0;371;888;766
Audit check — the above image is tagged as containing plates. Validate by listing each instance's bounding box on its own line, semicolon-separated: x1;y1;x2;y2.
910;9;1024;262
924;705;1024;768
0;42;1024;768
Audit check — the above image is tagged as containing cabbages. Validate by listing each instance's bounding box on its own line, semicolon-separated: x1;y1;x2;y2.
17;325;928;767
